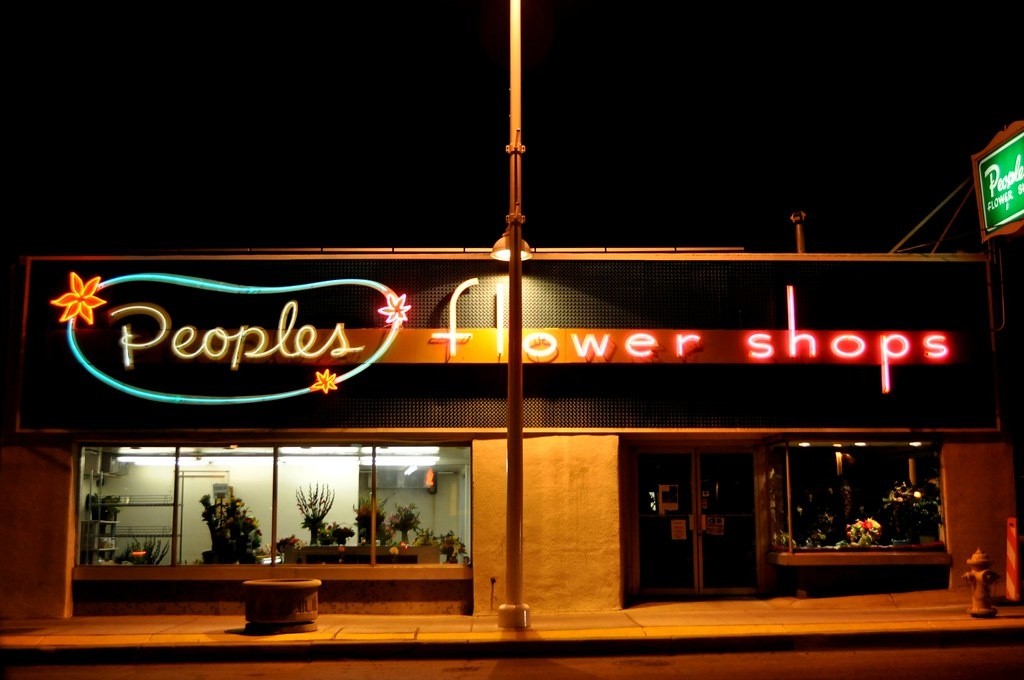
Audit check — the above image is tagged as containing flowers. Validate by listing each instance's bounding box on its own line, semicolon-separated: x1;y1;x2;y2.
199;485;262;550
270;534;308;554
295;480;335;531
846;517;882;547
438;530;467;558
352;492;392;546
388;502;422;532
882;480;944;526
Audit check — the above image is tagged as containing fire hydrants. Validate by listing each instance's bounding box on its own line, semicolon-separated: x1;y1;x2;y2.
962;546;999;618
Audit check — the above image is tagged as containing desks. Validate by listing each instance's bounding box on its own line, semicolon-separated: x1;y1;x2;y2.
283;546;440;564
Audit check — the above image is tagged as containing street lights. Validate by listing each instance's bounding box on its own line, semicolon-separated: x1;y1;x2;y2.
492;214;537;626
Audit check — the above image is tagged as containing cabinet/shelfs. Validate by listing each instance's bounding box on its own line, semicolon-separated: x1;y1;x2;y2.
80;520;119;564
85;469;184;566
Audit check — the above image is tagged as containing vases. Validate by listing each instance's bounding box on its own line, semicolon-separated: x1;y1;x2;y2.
402;531;409;544
446;554;458;564
309;527;318;546
239;552;256;564
891;538;911;545
